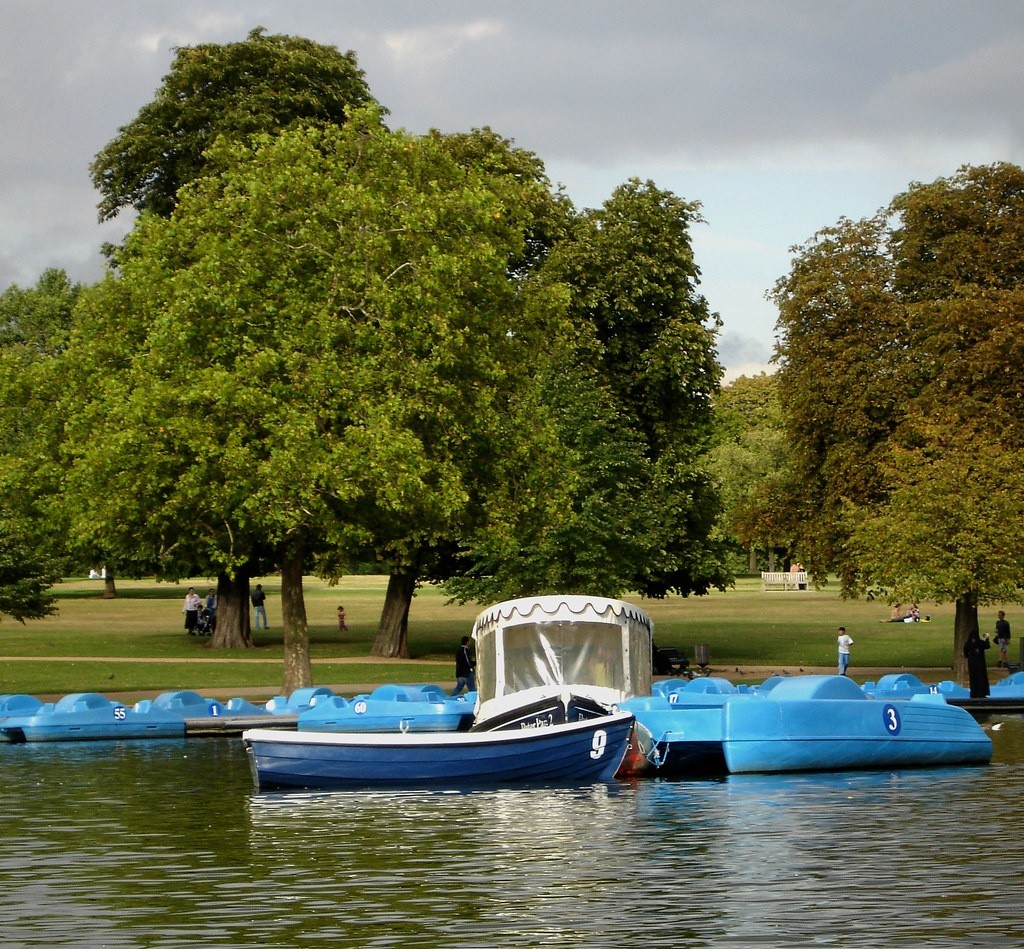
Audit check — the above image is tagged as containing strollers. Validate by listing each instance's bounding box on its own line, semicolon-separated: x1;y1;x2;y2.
192;607;214;636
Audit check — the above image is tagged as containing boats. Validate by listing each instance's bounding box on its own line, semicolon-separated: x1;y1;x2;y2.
653;670;1024;721
466;594;654;733
243;710;637;790
0;683;477;744
610;673;994;778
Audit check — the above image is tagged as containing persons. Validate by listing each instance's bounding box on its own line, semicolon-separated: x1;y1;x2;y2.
336;605;349;632
879;602;931;623
652;638;681;676
963;627;991;699
837;626;854;675
993;610;1011;668
251;584;270;630
450;636;477;696
183;587;218;638
790;562;807;590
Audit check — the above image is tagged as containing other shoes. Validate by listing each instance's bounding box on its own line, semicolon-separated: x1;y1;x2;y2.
264;627;269;629
1002;662;1008;667
995;661;1003;667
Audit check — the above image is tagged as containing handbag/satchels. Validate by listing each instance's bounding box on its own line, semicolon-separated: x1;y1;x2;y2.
993;636;998;644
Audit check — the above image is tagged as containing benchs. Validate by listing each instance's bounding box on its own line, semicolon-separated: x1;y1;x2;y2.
657;646;691;674
760;571;809;591
